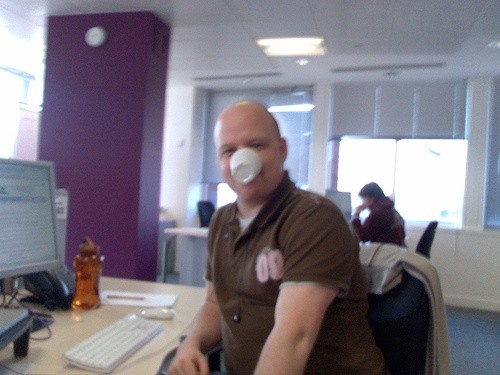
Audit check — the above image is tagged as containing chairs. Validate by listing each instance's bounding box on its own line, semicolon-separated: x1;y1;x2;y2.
415;221;437;258
353;243;448;375
199;200;217;228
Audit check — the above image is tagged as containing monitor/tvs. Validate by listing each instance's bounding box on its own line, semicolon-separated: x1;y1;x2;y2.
0;158;64;336
325;191;351;220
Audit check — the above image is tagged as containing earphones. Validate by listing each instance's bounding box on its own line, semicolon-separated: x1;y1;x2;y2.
232;307;241;321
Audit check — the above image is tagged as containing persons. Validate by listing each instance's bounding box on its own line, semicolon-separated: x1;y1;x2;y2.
350;182;406;246
168;99;387;375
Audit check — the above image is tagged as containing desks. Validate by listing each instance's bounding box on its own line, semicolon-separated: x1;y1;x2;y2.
0;269;209;375
164;225;210;286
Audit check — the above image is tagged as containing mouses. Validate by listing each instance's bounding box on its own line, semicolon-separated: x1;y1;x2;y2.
141;308;176;320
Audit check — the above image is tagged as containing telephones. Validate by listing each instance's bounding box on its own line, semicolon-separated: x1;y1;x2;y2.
20;263;76;311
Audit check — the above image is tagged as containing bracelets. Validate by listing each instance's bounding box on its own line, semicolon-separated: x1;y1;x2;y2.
352;213;359;217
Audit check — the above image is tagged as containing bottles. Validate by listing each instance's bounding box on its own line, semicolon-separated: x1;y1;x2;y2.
72;238;104;309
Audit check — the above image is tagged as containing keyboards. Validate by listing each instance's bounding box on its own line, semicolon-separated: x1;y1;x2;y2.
64;313;165;373
